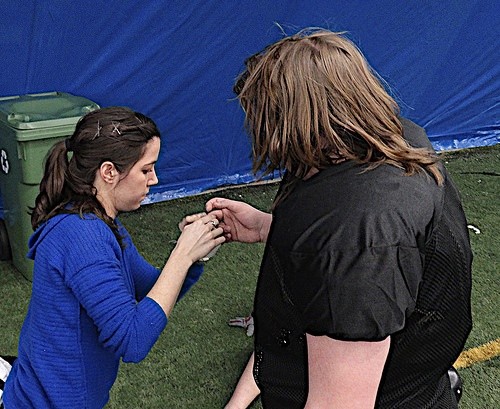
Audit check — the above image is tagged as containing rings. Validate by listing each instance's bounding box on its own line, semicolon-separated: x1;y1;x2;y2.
206;217;219;228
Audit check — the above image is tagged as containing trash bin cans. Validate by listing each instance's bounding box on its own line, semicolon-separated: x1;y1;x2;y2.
0;90;105;283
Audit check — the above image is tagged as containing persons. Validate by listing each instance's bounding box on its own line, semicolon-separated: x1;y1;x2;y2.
1;104;228;408
205;23;473;409
222;310;462;409
